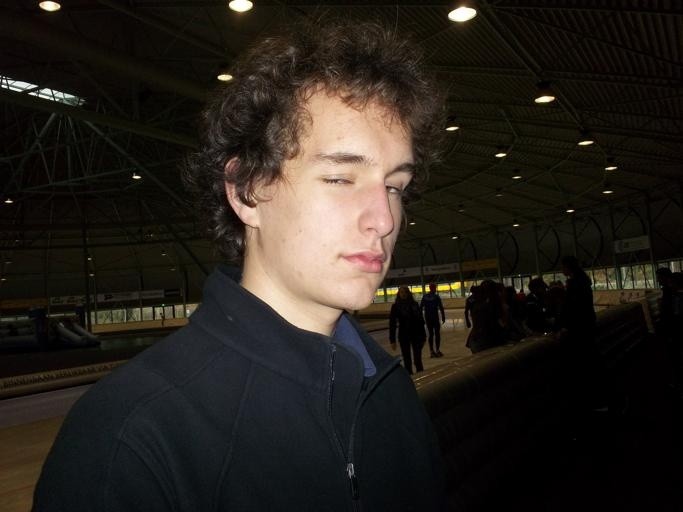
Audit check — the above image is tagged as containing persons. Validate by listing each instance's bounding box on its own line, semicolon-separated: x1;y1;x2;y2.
465;254;598;355
419;282;445;358
387;285;427;374
30;13;462;511
653;265;679;307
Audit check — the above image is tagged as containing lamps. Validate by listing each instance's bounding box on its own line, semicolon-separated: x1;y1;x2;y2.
146;230;154;237
409;219;415;225
604;159;618;171
132;171;141;179
497;190;503;197
446;123;460;132
39;0;60;11
495;150;507;157
513;173;522;179
576;133;594;146
458;207;463;213
452;235;457;240
229;0;254;13
567;208;574;212
601;187;615;194
531;77;557;106
513;222;520;227
218;73;233;81
448;4;477;23
1;74;87;107
